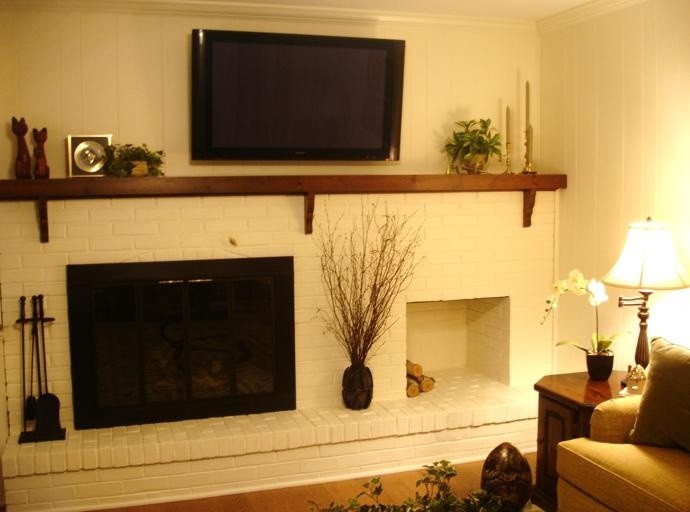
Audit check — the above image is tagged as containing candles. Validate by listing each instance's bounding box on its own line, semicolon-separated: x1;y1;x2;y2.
506;105;510;143
528;125;533;160
526;80;529;139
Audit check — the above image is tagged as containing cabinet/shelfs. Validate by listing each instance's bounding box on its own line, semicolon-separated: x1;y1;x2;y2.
530;370;628;512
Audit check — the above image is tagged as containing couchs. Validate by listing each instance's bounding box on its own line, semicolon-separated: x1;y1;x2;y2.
556;395;689;511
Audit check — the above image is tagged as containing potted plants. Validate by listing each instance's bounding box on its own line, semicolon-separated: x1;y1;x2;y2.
439;118;504;175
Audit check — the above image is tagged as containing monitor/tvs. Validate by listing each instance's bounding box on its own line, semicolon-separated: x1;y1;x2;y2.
191;29;405;161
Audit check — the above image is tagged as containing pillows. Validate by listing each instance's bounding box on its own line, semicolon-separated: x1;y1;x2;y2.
623;337;690;453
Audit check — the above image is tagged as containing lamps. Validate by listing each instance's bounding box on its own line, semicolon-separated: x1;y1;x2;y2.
601;214;690;388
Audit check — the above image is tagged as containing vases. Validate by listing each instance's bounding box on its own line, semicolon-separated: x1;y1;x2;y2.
341;367;373;410
127;161;148;176
586;357;614;380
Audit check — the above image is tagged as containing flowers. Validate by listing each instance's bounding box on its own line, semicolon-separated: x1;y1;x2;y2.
540;269;632;356
101;143;165;176
